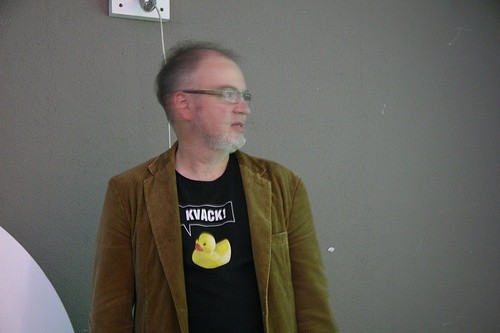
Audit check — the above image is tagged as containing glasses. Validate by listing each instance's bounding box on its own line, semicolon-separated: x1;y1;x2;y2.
183;90;252;104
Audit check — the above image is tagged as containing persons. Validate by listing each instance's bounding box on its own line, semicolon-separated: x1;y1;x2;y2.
90;42;338;333
